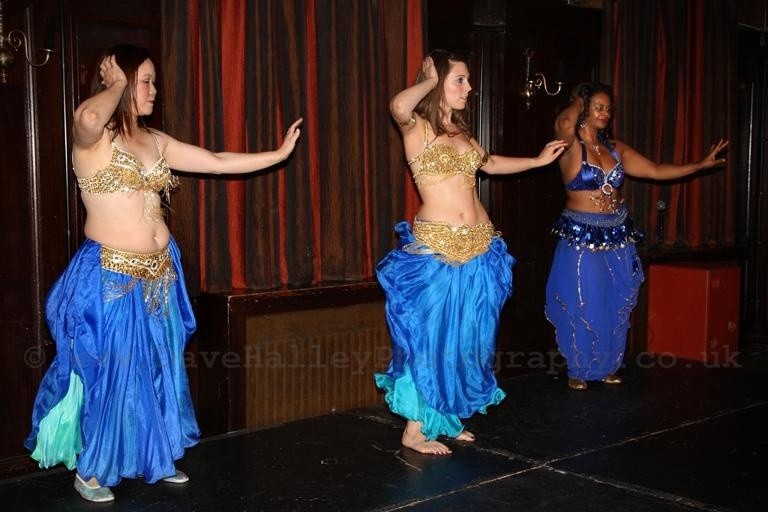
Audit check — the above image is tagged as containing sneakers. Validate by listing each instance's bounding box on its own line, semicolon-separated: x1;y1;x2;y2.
159;468;190;484
567;378;588;390
603;373;622;384
72;472;115;502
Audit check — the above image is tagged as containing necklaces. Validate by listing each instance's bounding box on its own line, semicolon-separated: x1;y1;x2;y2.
585;140;603;156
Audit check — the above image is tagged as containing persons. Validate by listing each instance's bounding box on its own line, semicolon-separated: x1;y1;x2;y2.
542;81;733;391
372;50;569;458
29;48;303;504
